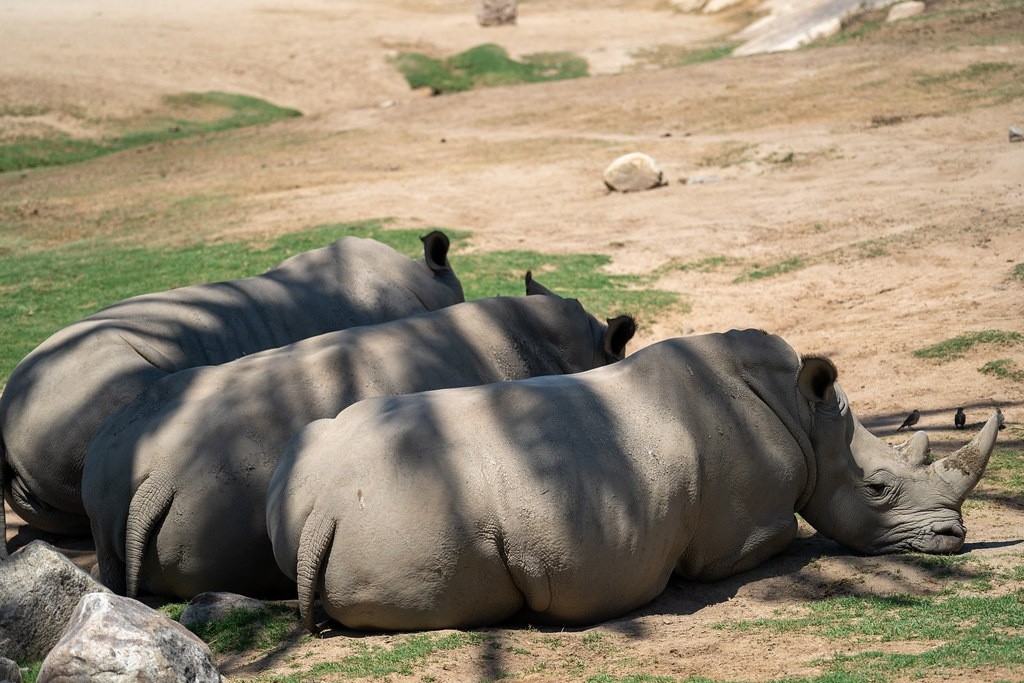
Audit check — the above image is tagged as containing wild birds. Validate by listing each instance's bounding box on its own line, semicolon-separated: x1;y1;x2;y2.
954;407;966;428
896;409;920;432
